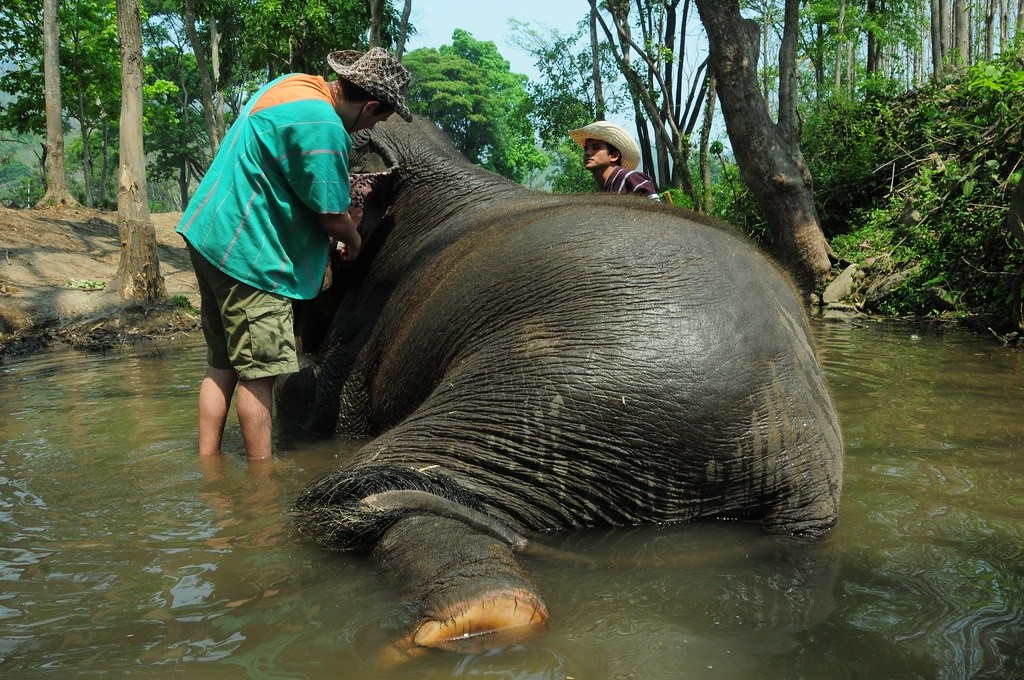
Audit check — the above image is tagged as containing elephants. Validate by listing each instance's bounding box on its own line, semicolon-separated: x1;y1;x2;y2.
270;107;846;677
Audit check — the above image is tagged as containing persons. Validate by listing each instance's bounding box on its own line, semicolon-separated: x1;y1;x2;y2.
174;46;415;461
568;120;663;204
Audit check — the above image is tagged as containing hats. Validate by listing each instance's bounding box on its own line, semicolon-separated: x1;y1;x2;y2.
567;121;640;171
327;45;414;123
